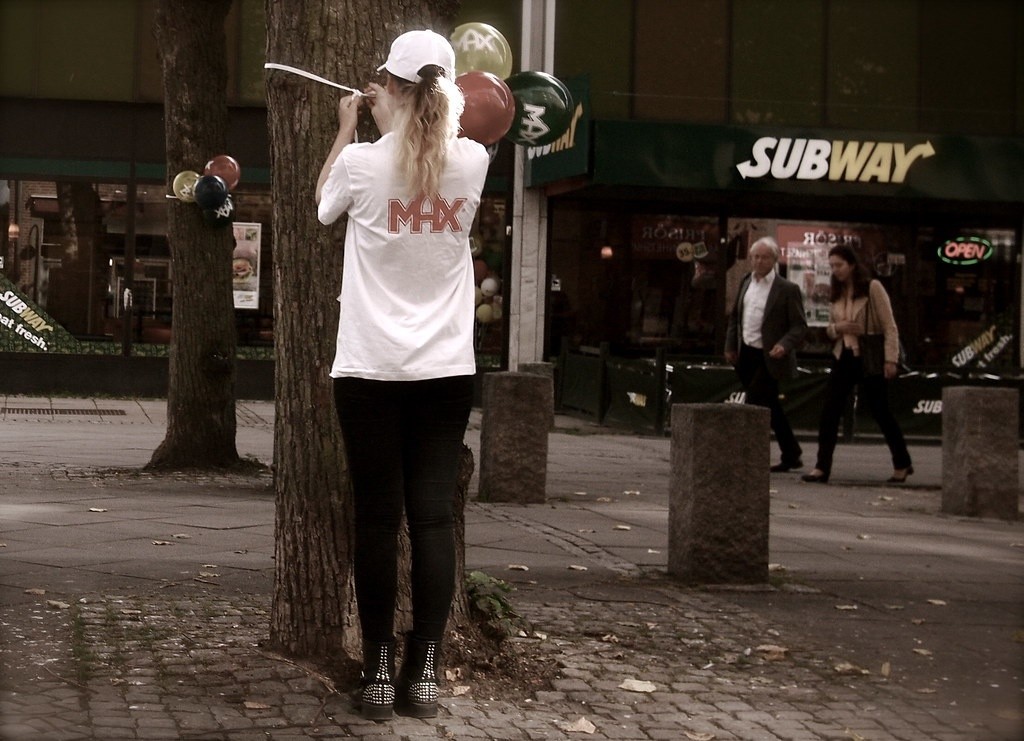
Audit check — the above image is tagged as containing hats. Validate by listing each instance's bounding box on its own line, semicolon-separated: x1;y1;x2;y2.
377;29;455;83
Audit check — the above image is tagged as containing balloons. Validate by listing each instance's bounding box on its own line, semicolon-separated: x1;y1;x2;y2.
172;156;240;231
450;22;573;148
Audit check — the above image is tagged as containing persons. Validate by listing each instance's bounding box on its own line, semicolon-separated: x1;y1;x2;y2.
802;245;913;484
725;236;808;472
316;30;489;719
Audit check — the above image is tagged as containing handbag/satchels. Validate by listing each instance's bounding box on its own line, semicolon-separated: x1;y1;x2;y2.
859;277;912;377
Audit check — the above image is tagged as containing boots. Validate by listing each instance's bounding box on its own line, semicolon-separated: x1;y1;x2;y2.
338;636;397;719
394;630;443;717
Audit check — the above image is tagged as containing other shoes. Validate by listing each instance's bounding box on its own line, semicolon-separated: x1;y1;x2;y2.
887;466;914;482
802;470;831;483
771;455;804;472
